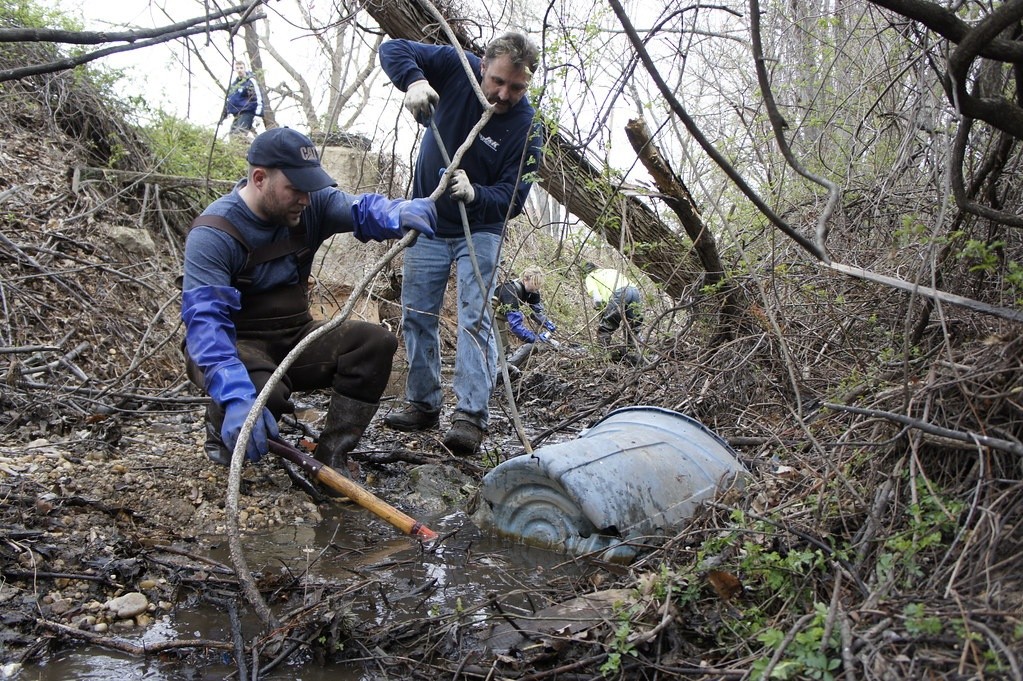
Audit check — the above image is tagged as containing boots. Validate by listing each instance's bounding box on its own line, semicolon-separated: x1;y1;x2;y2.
308;388;381;504
204;399;232;466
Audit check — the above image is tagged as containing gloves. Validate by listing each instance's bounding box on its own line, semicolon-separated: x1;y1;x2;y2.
507;310;548;344
351;193;438;248
529;303;555;332
404;80;440;128
181;284;279;463
438;167;475;205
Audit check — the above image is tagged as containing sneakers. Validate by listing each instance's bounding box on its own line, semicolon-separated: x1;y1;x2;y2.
443;420;483;454
385;404;441;432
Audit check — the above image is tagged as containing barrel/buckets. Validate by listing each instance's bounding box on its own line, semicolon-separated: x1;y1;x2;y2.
469;405;751;562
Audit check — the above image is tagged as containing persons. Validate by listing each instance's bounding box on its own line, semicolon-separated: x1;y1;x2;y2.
582;263;643;346
378;33;545;451
180;126;439;501
225;60;259;141
491;265;556;348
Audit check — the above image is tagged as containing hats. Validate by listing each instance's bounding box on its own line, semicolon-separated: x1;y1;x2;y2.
246;126;338;191
